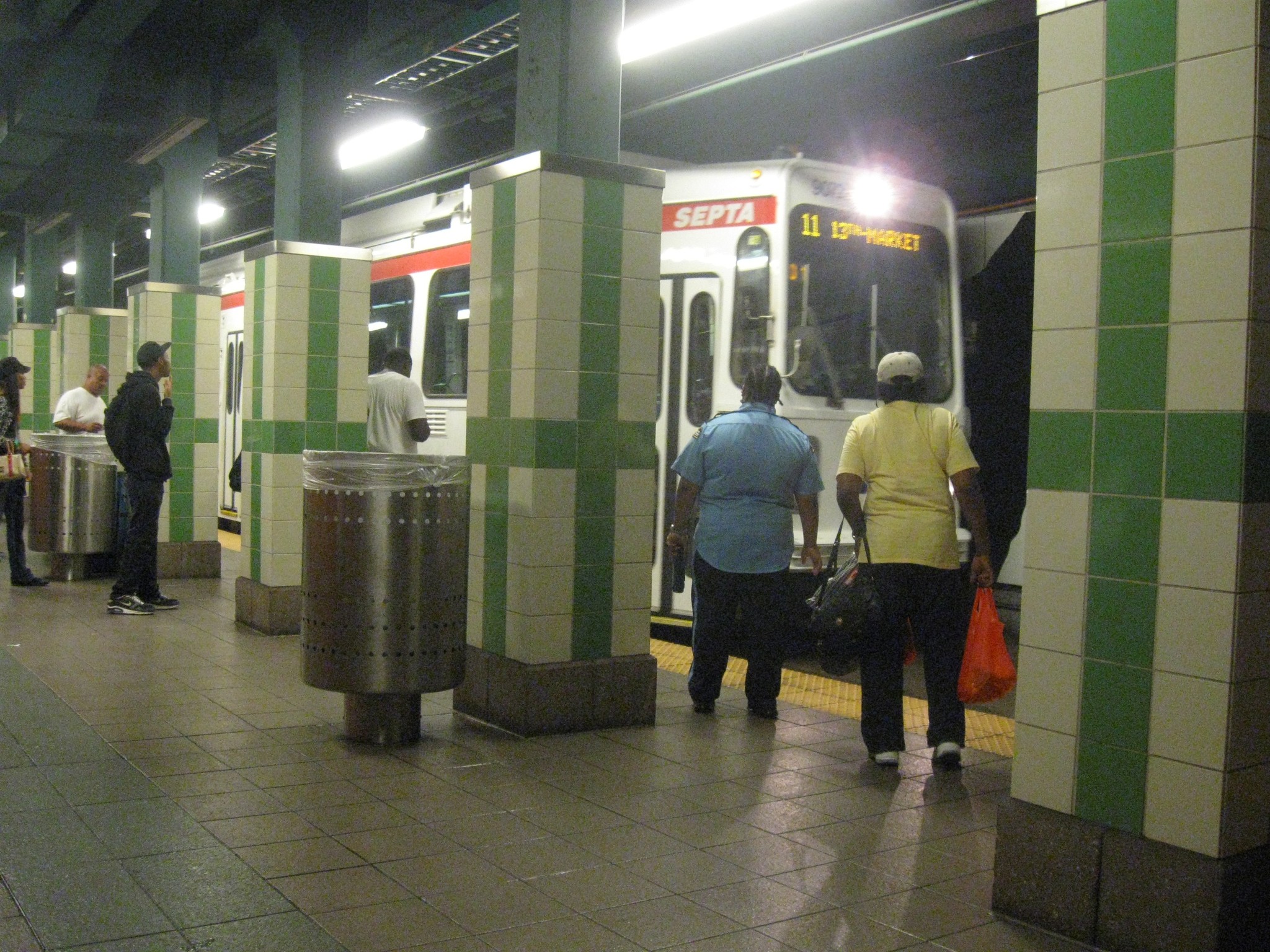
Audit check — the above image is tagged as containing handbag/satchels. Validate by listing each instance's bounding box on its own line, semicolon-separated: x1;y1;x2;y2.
0;440;31;481
795;511;880;675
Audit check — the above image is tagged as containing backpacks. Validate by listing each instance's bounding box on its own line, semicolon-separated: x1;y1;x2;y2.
104;379;153;471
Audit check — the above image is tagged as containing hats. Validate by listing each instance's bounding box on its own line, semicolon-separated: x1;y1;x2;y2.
136;340;171;368
0;357;31;379
876;350;925;387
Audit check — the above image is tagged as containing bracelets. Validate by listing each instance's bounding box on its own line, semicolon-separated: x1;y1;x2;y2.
14;440;18;447
670;524;684;534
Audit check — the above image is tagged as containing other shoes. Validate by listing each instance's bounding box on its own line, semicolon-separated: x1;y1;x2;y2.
957;586;1014;706
28;577;49;586
693;700;716;713
749;705;778;718
932;742;961;763
867;752;899;765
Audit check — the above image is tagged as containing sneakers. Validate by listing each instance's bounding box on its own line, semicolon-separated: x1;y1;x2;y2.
107;594;155;616
144;593;179;610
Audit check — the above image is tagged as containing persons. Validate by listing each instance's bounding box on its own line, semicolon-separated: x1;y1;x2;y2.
836;350;994;763
52;364;109;432
368;348;430;454
664;363;824;719
106;341;180;615
0;357;50;586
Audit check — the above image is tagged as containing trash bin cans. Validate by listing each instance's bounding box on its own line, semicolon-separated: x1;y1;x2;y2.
27;436;119;557
299;448;472;694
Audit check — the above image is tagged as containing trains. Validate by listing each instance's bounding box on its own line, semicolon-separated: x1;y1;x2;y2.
211;157;973;630
